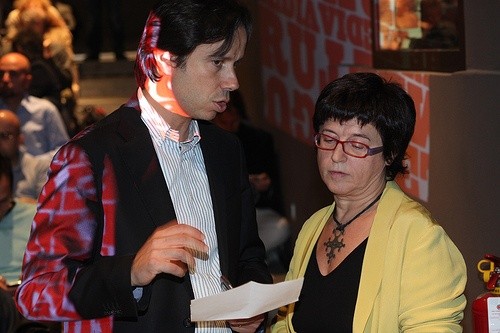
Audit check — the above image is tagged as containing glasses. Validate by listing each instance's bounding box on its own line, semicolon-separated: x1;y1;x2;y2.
0;132;16;139
314;133;384;158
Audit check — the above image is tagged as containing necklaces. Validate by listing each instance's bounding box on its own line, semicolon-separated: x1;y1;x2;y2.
332;192;382;231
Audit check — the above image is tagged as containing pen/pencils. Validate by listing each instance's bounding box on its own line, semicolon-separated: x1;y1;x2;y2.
220;276;233;290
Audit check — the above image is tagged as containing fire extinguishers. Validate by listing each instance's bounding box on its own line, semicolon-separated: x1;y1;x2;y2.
471;254;500;333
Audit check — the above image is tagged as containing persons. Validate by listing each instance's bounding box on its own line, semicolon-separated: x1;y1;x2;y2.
0;0;78;333
15;0;287;333
271;72;468;333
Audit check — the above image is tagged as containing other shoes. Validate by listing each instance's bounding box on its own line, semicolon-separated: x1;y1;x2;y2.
72;84;80;97
